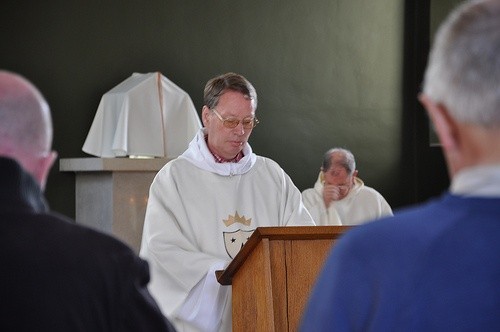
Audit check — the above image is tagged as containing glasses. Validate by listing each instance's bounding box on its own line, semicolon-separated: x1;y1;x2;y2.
212;108;260;129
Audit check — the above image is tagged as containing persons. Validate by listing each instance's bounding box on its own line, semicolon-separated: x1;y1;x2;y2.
294;0;500;332
139;72;317;332
0;71;177;332
301;147;395;226
